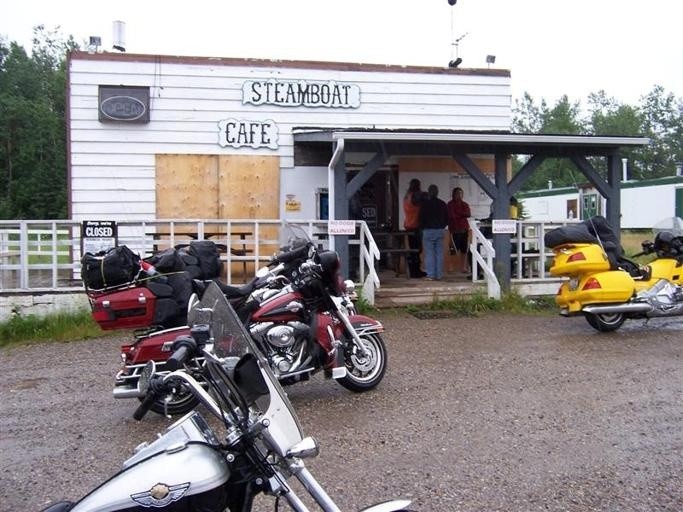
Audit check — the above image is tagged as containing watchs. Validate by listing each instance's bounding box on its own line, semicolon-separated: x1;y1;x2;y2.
39;281;418;512
544;170;683;333
80;224;388;416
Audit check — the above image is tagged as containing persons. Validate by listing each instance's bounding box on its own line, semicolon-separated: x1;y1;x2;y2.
403;179;429;278
447;187;471;273
418;184;448;281
509;197;518;219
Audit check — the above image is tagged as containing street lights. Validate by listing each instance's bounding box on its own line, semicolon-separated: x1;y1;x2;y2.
79;246;143;293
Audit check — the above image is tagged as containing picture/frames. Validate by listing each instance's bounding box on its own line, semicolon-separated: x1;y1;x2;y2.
370;232;416;280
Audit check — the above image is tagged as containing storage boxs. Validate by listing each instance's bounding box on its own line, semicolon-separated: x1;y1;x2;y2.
373;248;422;280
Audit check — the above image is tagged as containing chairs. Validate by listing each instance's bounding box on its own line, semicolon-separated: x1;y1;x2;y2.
414;271;427;278
424;276;436;281
